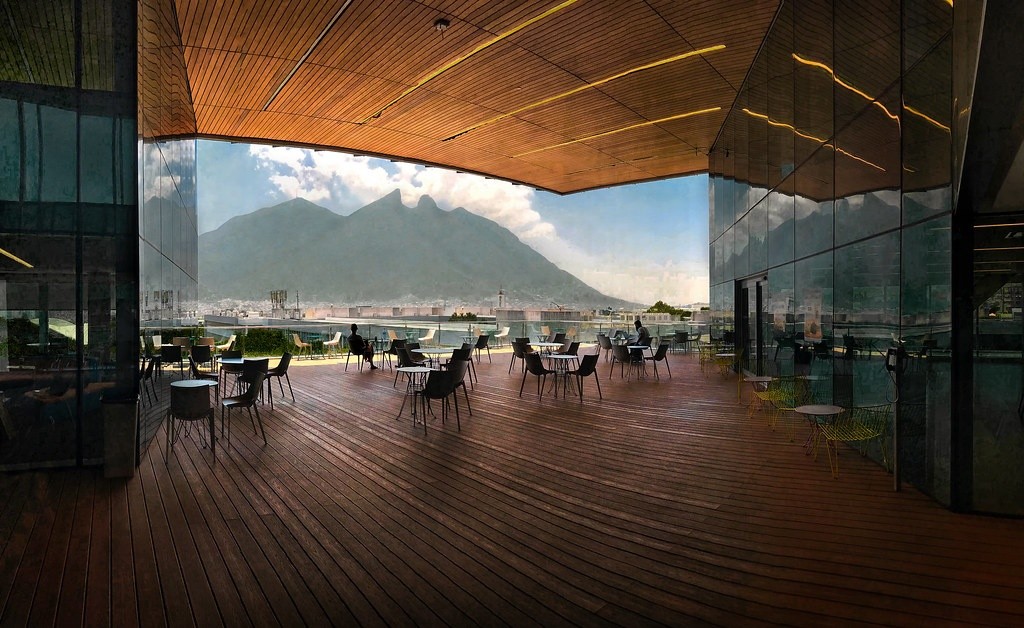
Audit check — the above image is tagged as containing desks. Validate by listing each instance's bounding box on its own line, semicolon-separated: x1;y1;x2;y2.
715;353;736;376
366;339;391;370
411;348;455;370
609;338;626;364
794;405;846;456
216;358;244;398
743;376;780;410
547;355;577;397
625;346;649;382
171;380;218;449
699;345;716;361
526;343;565;370
26;381;116;433
666;332;691;355
396;367;437;428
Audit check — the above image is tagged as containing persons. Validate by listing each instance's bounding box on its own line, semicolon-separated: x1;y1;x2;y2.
347;324;377;370
630;320;651;358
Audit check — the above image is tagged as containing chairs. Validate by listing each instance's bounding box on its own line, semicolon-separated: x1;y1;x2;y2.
133;335;195;407
165;325;509;464
697;340;892;479
509;326;702;403
